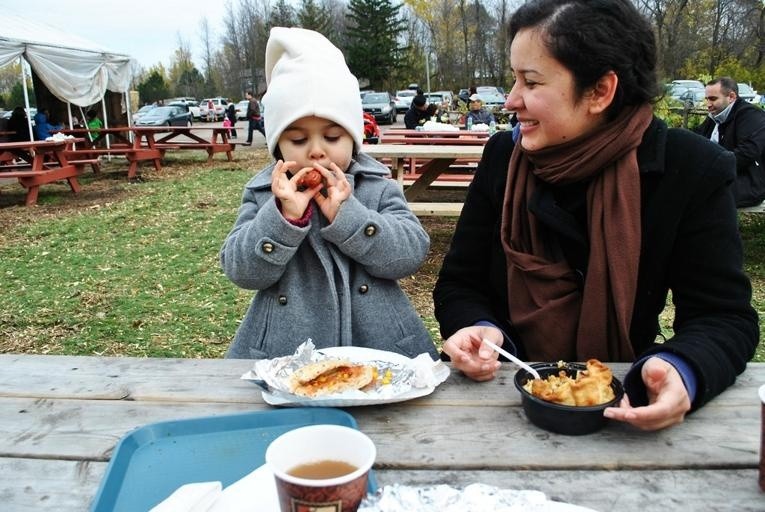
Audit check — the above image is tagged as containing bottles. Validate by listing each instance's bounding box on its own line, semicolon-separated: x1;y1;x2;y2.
467;117;474;131
488;117;496;136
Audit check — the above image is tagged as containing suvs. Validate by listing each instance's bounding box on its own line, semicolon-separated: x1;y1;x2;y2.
459;86;506;112
394;90;417;114
168;101;200;120
199;97;228;121
422;90;457;110
362;93;397;124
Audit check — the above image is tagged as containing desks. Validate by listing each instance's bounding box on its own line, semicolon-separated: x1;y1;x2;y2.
0;137;84;208
53;126;173;178
1;354;764;512
360;144;491;200
133;125;244;164
384;124;491;145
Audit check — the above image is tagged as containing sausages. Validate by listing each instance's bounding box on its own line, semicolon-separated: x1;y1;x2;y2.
295;171;322;188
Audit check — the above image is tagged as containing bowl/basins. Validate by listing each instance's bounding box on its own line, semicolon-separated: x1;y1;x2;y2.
514;361;625;436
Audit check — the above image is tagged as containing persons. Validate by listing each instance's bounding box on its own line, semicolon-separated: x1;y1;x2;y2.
219;25;442;362
5;107;31;142
432;0;761;431
85;109;104;145
690;77;761;209
70;106;80;127
33;109;66;140
465;95;495;130
242;91;268;146
405;94;439;130
222;98;237;139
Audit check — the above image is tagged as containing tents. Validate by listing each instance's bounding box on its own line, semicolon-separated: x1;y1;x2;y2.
0;38;133;164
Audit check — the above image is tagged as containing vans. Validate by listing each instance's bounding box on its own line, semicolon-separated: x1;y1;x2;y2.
671;80;705;88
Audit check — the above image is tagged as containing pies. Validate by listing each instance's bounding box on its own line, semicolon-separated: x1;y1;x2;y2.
289;358;375;397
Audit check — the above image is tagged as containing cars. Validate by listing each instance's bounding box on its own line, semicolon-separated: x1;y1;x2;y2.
237;101;263;121
134;106;193;132
736;84;758;104
676;87;709;110
132;105;158;124
662;83;691;101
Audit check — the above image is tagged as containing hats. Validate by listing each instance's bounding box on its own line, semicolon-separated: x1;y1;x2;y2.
86;109;97;119
260;27;365;159
469;94;483;101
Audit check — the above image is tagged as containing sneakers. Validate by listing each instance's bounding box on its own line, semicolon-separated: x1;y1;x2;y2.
242;143;252;145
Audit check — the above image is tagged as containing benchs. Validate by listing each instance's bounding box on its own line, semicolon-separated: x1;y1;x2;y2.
2;158;100;170
399;201;465;217
63;148;142;155
178;141;214;149
108;141;179;151
0;165;43;180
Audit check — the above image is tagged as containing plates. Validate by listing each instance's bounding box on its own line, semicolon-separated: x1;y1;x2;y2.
261;344;437;407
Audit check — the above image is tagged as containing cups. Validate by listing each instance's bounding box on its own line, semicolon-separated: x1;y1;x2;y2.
265;424;377;512
